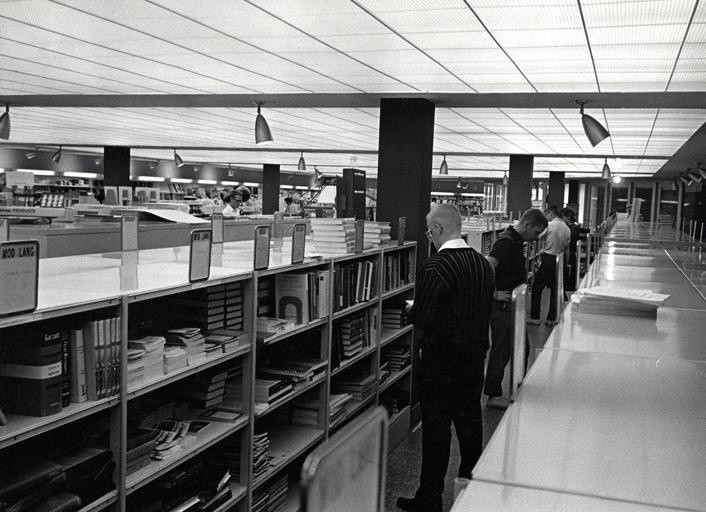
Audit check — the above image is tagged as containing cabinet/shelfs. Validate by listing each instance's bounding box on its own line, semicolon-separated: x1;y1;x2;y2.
0;183;522;512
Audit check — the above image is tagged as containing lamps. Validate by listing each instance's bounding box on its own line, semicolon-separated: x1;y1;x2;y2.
0;103;15;140
249;98;275;144
439;152;448;175
670;161;705;191
573;98;611;148
296;151;306;171
4;144;235;176
314;165;322;180
600;154;612;180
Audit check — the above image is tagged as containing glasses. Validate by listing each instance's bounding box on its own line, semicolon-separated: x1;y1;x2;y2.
231;198;242;202
424;225;436;241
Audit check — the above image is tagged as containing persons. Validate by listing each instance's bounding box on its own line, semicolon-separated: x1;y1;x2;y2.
222;192;243;219
562;207;579;299
397;204;496;512
485;208;549;409
284;197;299;216
525;205;571;326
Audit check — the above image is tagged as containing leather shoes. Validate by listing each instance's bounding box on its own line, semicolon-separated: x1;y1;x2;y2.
545;320;555;327
396;496;443;512
526;318;540;326
487;396;510;409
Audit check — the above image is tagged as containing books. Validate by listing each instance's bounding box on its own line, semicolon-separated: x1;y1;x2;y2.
578;286;672;319
483;234;492;256
